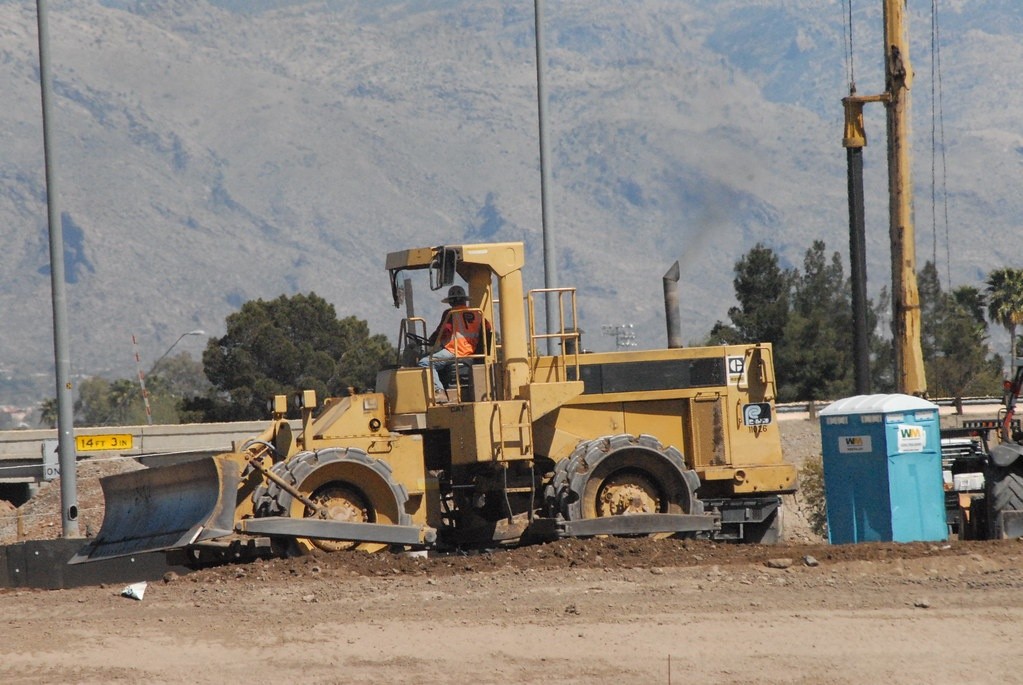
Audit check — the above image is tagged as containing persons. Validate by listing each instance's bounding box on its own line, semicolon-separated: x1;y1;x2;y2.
418;285;491;403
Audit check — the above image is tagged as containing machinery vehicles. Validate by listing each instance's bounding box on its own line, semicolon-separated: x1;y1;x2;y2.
841;0;1023;544
64;242;797;571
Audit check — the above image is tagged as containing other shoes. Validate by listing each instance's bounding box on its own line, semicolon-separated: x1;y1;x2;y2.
435;389;448;402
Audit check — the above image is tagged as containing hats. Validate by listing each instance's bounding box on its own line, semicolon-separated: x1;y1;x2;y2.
440;286;473;303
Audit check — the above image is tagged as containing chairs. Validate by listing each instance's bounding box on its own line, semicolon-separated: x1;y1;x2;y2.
446;329;490;371
